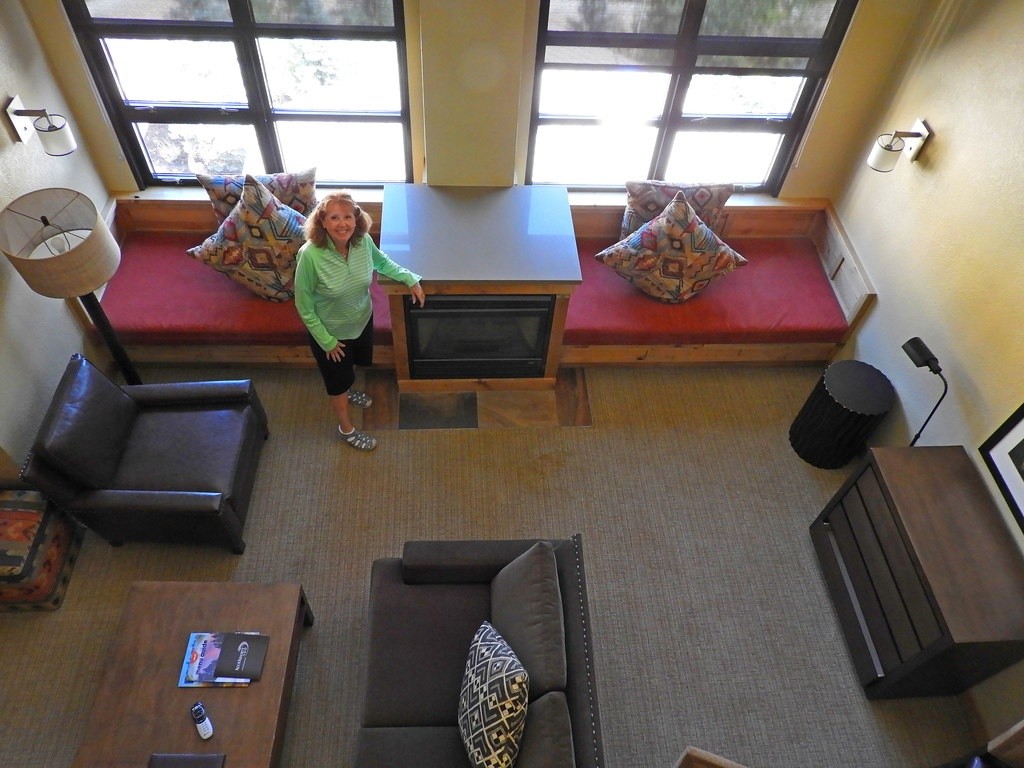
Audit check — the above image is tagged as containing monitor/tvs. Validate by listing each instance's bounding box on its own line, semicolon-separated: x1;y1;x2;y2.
411;308;547;359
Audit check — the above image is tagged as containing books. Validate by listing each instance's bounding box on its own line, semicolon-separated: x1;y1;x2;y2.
178;631;270;688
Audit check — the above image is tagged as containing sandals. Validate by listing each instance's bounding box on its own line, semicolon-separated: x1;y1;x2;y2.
336;424;377;452
346;387;372;409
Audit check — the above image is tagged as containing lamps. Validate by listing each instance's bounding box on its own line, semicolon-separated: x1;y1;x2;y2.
0;188;144;384
867;118;932;173
5;93;79;156
901;337;949;446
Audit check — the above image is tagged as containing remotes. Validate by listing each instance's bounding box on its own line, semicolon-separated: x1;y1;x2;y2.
191;702;213;739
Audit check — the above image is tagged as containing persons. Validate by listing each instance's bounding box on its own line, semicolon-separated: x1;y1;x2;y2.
293;189;427;451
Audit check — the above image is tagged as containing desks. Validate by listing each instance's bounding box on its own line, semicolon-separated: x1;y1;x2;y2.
810;448;1022;701
377;183;582;391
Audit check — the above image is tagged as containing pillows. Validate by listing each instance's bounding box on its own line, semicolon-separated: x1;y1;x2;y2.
619;182;734;245
593;190;747;304
456;619;529;768
186;173;308;302
195;168;319;234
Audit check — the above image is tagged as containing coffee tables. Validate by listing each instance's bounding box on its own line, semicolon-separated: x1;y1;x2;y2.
75;582;313;768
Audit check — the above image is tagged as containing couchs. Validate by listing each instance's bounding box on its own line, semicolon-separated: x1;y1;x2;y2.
358;534;604;768
76;195;394;369
565;200;877;364
16;352;269;554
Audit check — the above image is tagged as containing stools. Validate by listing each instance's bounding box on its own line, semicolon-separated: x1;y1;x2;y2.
0;488;85;611
